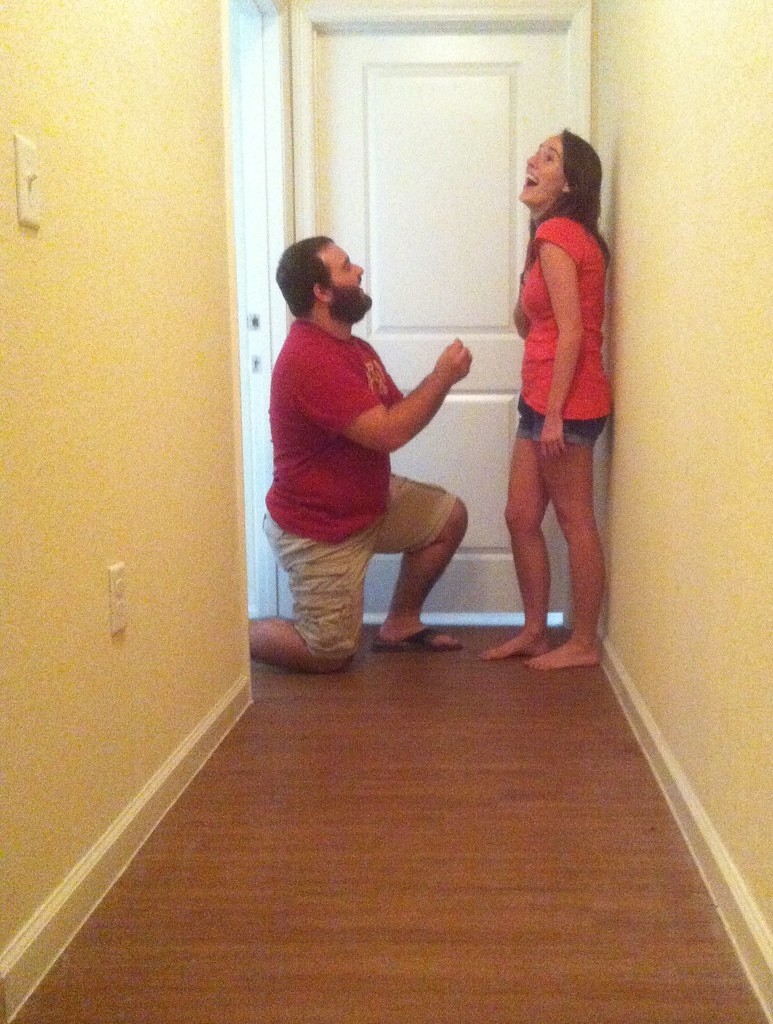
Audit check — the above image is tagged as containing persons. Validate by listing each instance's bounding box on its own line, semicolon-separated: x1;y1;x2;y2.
476;127;611;669
248;234;473;675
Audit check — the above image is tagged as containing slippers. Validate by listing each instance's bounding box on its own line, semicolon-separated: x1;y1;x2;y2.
371;626;464;654
